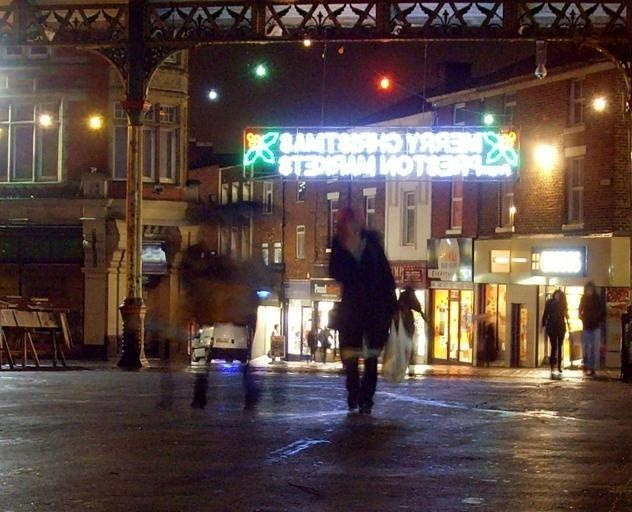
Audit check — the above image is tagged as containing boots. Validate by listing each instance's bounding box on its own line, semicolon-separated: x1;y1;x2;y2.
189;376;208;409
358;357;378;415
241;365;262;407
342;355;359;411
551;357;562;379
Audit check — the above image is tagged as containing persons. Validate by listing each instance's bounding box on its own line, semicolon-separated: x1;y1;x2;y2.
396;282;427;380
539;288;572;380
268;321;333;364
580;279;606;376
478;320;498;368
326;201;398;415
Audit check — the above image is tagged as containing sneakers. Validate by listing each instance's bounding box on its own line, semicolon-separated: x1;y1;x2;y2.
583;364;595;376
